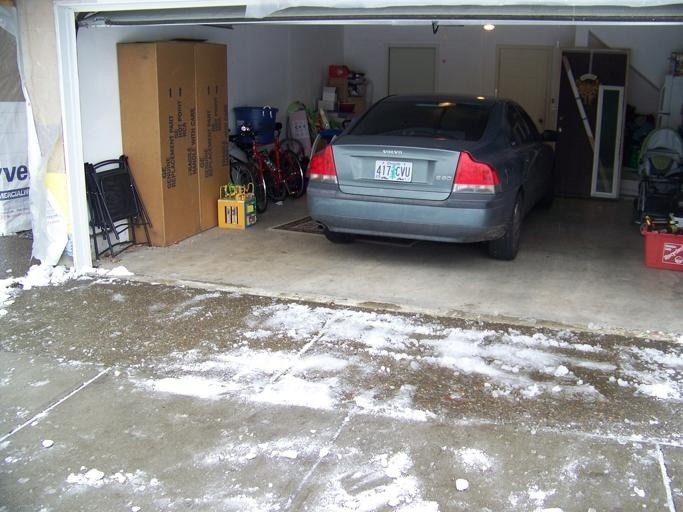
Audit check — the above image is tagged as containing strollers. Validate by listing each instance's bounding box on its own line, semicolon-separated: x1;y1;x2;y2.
634;127;682;235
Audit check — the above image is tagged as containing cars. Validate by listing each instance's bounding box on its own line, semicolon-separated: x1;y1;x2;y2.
298;93;560;264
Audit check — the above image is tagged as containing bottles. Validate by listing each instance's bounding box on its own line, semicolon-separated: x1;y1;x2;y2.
645;212;682;234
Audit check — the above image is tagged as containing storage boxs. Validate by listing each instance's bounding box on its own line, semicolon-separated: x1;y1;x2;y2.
640;223;683;272
328;76;366;117
117;40;229;248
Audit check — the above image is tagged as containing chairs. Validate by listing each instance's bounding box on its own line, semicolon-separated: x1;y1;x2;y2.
85;154;153;259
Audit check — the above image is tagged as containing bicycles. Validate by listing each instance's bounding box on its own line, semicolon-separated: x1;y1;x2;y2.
220;116;306;211
